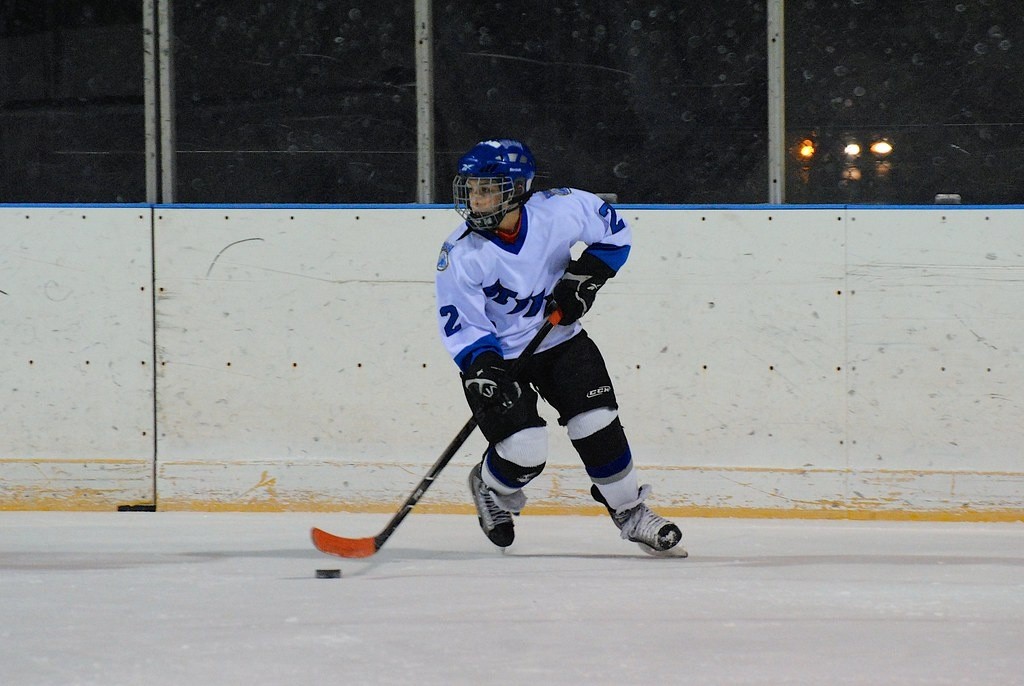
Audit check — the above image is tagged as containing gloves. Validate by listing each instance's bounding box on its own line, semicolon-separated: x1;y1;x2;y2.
463;352;522;414
544;252;615;326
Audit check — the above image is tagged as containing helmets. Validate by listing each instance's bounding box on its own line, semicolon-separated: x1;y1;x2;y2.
456;137;535;203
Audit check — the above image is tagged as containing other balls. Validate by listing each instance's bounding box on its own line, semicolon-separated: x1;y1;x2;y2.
315;568;342;580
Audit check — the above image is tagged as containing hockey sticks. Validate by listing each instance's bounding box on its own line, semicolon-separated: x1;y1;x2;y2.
308;308;566;560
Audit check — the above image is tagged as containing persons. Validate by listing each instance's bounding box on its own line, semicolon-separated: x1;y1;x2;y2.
435;142;689;559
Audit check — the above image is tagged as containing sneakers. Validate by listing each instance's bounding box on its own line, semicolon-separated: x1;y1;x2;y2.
591;484;688;558
468;446;528;556
